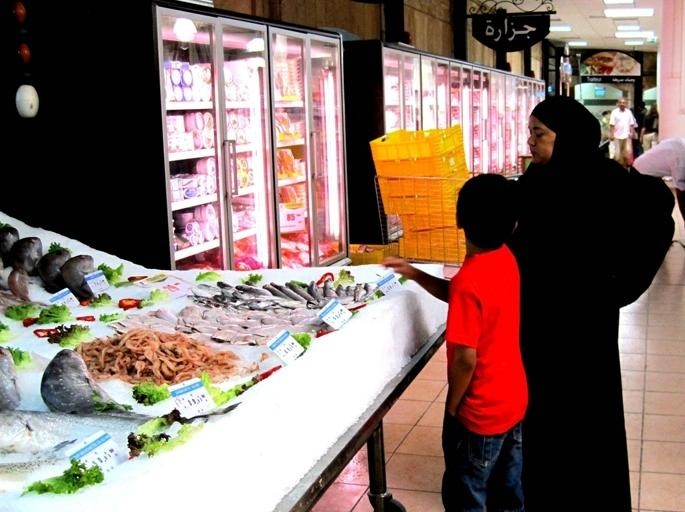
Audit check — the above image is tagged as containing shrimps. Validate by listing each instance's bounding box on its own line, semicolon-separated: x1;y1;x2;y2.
76;327;242;386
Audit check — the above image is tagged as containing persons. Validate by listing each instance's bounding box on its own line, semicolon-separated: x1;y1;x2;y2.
601;98;658;169
629;136;685;246
380;171;529;512
513;95;632;512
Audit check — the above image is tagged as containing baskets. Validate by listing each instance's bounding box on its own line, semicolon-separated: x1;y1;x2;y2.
369;124;467;263
349;245;397;265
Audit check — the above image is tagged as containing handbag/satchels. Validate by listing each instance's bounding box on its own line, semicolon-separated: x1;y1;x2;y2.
619;166;676;307
